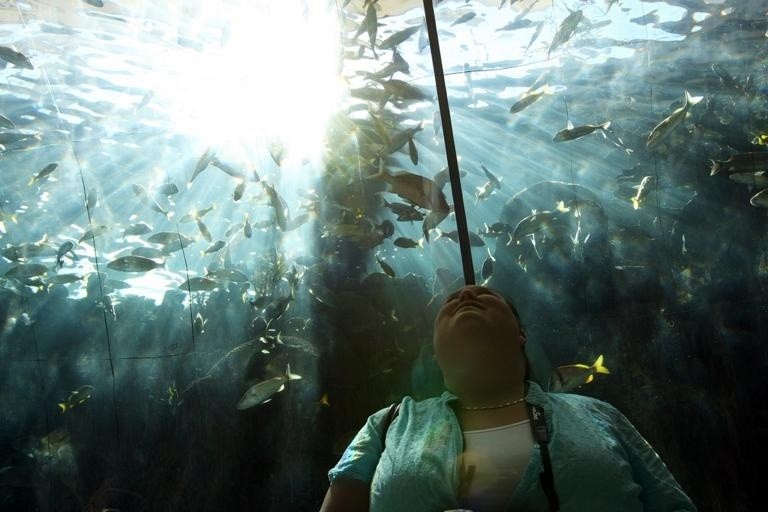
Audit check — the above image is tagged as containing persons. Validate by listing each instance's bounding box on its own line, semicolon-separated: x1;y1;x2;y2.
319;285;698;512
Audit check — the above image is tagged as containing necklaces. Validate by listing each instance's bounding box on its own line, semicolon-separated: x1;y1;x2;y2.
458;398;525;410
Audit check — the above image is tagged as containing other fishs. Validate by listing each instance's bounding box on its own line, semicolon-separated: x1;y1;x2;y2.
0;0;763;511
